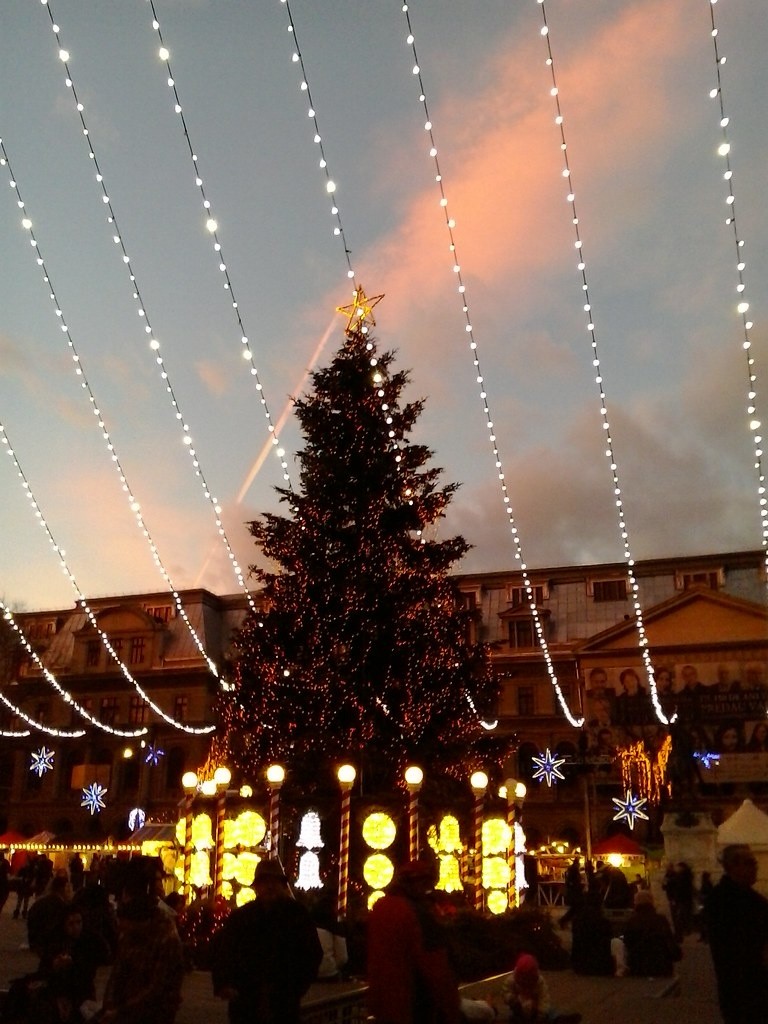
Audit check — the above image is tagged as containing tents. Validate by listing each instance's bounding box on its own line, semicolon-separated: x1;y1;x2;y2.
592;833;649;879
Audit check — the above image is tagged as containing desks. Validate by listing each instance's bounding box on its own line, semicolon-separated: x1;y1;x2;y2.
538;881;566;907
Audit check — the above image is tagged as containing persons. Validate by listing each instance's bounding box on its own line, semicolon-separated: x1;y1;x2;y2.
310;885;349;984
211;860;323;1024
0;850;119;1024
588;727;636;757
714;722;768;752
710;663;740;690
619;668;647;697
695;842;768;1024
101;870;183;1024
742;661;768;690
500;954;550;1016
678;665;709;693
647;664;675;694
365;858;466;1024
459;997;582;1024
558;858;714;978
586;668;616;696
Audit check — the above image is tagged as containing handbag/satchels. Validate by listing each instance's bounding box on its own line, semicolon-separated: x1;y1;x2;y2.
610;935;633;977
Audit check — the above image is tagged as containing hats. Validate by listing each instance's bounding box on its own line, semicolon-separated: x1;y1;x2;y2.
250;859;288;887
514;954;539;985
